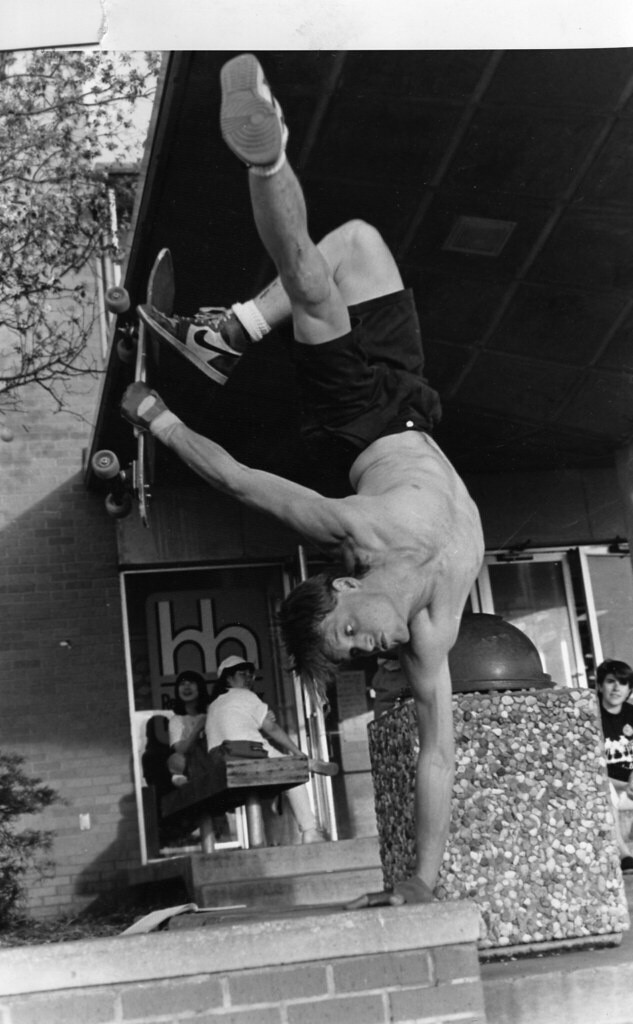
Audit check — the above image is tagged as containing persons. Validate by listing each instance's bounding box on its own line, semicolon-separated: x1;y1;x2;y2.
168;654;340;843
594;659;633;874
119;52;485;909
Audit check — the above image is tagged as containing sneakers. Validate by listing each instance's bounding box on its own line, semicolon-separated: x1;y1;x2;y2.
220;54;285;168
135;303;252;387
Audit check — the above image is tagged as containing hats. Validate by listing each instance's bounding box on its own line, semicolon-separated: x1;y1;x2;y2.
216;655;255;679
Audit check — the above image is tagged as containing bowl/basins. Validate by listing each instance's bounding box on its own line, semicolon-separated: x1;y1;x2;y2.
373;612;554;699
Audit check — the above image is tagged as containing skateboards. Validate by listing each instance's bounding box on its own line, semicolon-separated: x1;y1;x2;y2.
89;246;177;532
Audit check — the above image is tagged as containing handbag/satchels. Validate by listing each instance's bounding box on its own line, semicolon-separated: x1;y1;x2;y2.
209;740;269;768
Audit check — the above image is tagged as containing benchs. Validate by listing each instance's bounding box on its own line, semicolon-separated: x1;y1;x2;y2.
158;756;311;854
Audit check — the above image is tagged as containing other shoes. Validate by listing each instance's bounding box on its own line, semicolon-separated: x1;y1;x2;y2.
310;761;339;776
171;774;189;787
622;857;633;874
302;829;332;844
